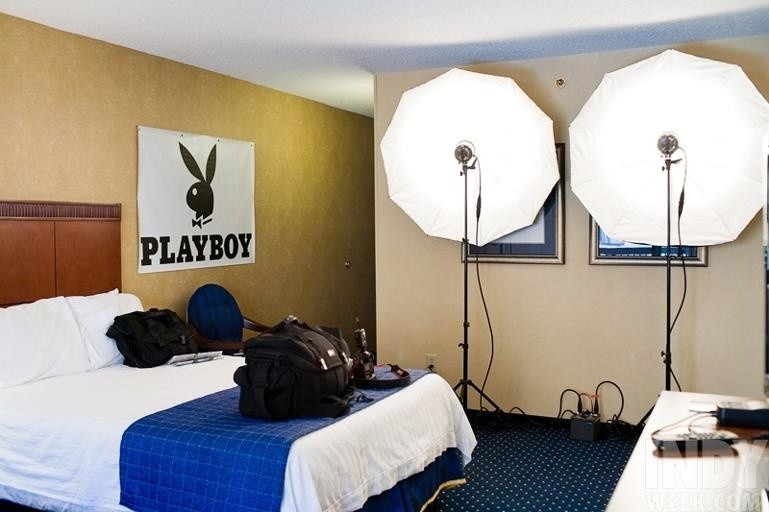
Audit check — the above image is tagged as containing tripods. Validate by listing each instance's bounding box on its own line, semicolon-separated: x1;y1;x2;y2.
452;162;516;431
623;158;678;441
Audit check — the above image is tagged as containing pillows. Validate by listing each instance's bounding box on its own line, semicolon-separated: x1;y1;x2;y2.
0;296;90;390
66;289;143;371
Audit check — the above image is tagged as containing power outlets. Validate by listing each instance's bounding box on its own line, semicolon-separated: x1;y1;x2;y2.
427;354;438;373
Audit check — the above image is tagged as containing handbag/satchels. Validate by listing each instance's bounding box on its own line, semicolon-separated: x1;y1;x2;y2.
105;307;198;368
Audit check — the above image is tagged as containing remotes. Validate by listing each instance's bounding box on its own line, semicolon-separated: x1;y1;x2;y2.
651;427;740;451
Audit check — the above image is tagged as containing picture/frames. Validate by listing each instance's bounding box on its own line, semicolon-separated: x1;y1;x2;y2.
461;142;566;265
589;214;708;268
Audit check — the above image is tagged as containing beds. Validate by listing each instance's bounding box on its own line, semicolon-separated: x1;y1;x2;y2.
0;201;478;512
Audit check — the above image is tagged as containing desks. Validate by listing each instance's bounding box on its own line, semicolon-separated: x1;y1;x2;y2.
606;389;768;512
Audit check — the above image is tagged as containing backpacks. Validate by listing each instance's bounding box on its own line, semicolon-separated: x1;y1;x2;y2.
232;319;352;423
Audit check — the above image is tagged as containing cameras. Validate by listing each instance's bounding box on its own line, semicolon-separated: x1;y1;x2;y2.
352;328;376;381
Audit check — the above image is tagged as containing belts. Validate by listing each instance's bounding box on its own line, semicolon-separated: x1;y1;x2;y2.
349;361;410;389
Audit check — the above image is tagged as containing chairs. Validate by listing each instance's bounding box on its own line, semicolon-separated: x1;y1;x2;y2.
185;283;273;356
